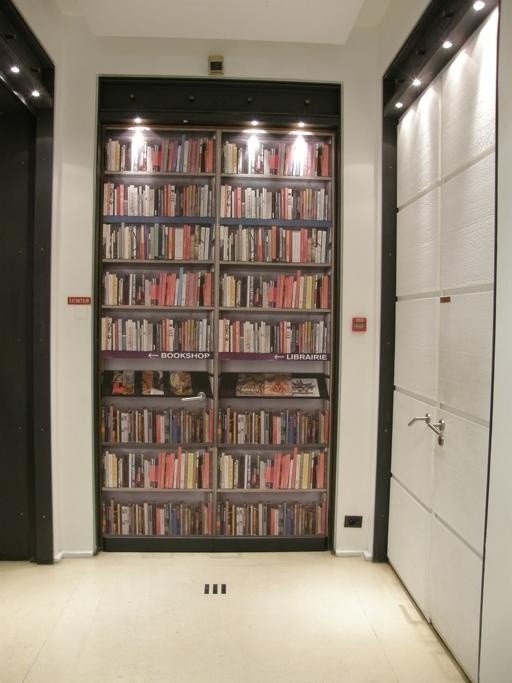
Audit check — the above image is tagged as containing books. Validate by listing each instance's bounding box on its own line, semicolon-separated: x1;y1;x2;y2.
101;222;329;355
99;371;331;540
101;127;332;215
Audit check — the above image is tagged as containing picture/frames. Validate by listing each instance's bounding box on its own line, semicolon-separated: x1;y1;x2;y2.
93;117;341;552
